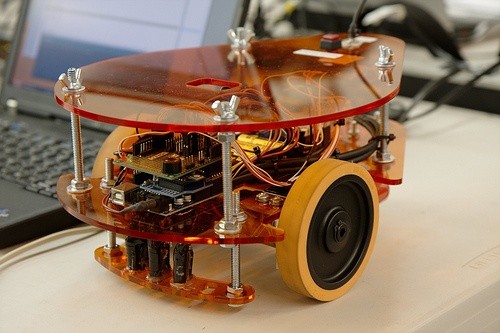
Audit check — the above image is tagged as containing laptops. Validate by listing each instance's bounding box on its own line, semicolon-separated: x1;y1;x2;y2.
0;0;249;249
